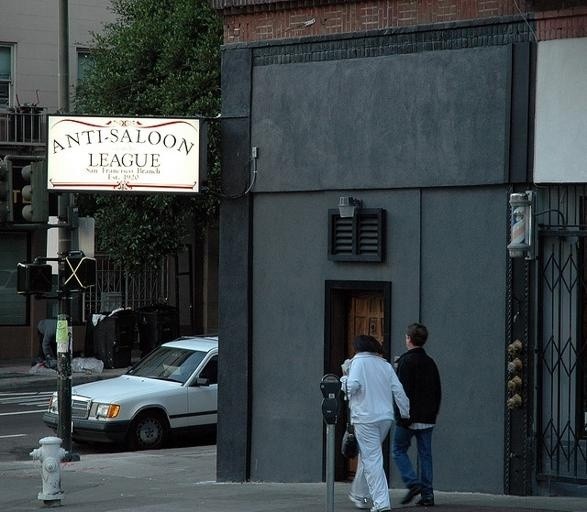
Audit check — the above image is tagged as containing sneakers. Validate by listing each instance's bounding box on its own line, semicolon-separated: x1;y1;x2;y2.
345;488;371;508
415;496;435;507
370;505;391;512
401;484;423;504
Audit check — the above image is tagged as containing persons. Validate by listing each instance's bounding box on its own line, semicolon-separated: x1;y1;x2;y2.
393;323;441;506
340;335;410;512
37;319;56;357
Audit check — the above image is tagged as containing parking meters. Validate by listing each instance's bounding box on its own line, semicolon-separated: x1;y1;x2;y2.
319;373;342;512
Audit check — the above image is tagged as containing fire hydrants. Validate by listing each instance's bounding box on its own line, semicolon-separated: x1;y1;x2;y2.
29;436;69;506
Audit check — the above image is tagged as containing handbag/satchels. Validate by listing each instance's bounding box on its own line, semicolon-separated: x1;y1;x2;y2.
341;430;359;459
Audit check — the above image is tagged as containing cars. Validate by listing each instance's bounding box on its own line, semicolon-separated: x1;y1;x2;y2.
42;335;218;451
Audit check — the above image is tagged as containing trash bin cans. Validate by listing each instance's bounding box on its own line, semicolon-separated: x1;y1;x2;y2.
88;303;180;369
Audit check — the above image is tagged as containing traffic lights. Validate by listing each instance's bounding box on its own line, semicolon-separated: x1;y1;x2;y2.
17;263;52;294
21;161;49;223
0;160;13;222
